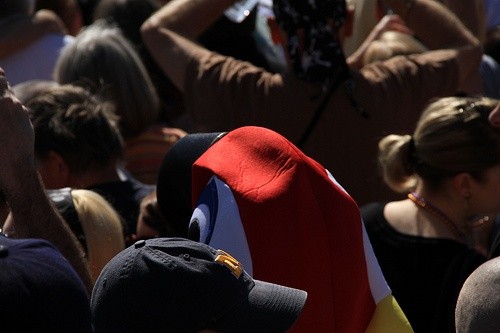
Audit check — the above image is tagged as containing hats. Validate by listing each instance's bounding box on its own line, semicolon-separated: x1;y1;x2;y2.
90;238;307;333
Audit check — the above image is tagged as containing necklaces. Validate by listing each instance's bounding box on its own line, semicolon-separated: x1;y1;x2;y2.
407;192;468;241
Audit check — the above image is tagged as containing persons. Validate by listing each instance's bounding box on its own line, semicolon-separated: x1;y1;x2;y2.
0;0;500;332
140;0;484;203
